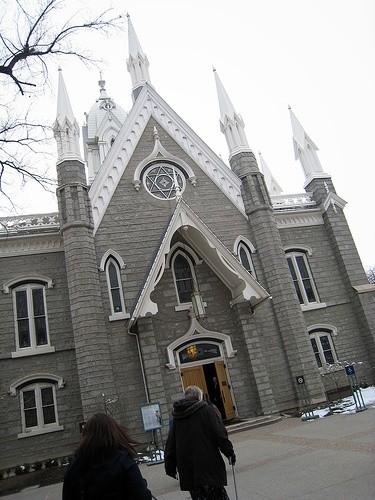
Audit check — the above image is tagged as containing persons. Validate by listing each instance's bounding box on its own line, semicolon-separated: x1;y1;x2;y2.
62;413;154;500
164;385;239;500
